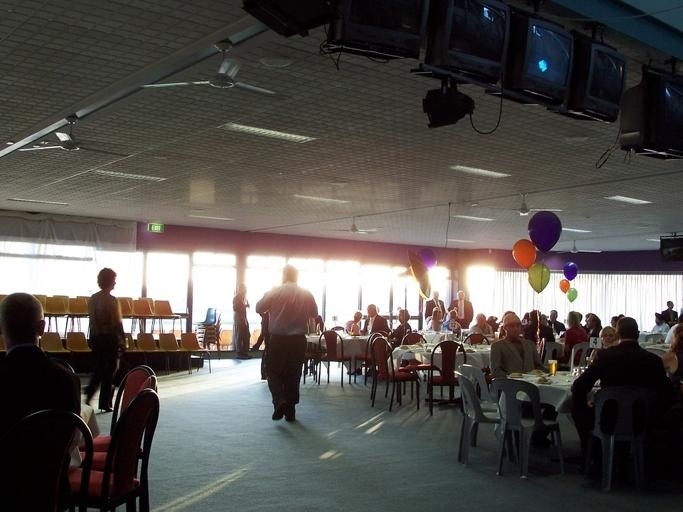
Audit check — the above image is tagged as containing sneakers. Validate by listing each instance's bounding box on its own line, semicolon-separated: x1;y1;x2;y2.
271;398;290;420
347;368;362;375
284;404;296;421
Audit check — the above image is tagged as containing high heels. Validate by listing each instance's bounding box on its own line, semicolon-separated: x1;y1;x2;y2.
97;404;115;413
82;385;93;406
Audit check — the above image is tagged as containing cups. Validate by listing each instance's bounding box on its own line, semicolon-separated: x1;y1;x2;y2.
547;360;557;376
494;332;498;342
571;365;589;380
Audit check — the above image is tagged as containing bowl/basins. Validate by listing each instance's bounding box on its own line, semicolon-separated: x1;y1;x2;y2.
510;373;522;377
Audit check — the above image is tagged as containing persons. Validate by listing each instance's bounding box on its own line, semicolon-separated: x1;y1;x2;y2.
425;291;446;326
571;318;668;467
230;265;326;421
447;290;473;329
0;293;81;512
84;267;129;413
651;301;682;389
344;304;626;449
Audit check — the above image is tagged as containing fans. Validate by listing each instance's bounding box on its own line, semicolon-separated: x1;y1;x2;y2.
16;111;129;157
134;37;280;97
484;191;566;216
550;238;605;254
319;215;382;235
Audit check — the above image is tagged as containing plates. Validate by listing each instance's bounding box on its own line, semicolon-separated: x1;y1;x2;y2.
521;374;549;377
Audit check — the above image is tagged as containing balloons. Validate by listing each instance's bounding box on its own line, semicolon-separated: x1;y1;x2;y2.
417;245;439;268
512;210;579;301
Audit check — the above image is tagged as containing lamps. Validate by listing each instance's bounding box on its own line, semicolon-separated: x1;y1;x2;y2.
416;77;477;131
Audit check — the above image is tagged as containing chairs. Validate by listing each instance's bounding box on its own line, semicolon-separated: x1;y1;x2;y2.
33;293;224;378
304;311;488;418
68;364;162;512
302;307;683;493
2;406;96;512
454;313;682;494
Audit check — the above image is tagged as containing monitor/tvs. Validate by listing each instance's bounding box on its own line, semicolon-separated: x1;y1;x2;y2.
546;35;627;123
410;1;511;93
659;237;683;262
485;17;576;111
239;1;340;39
328;1;423;63
595;71;683;169
